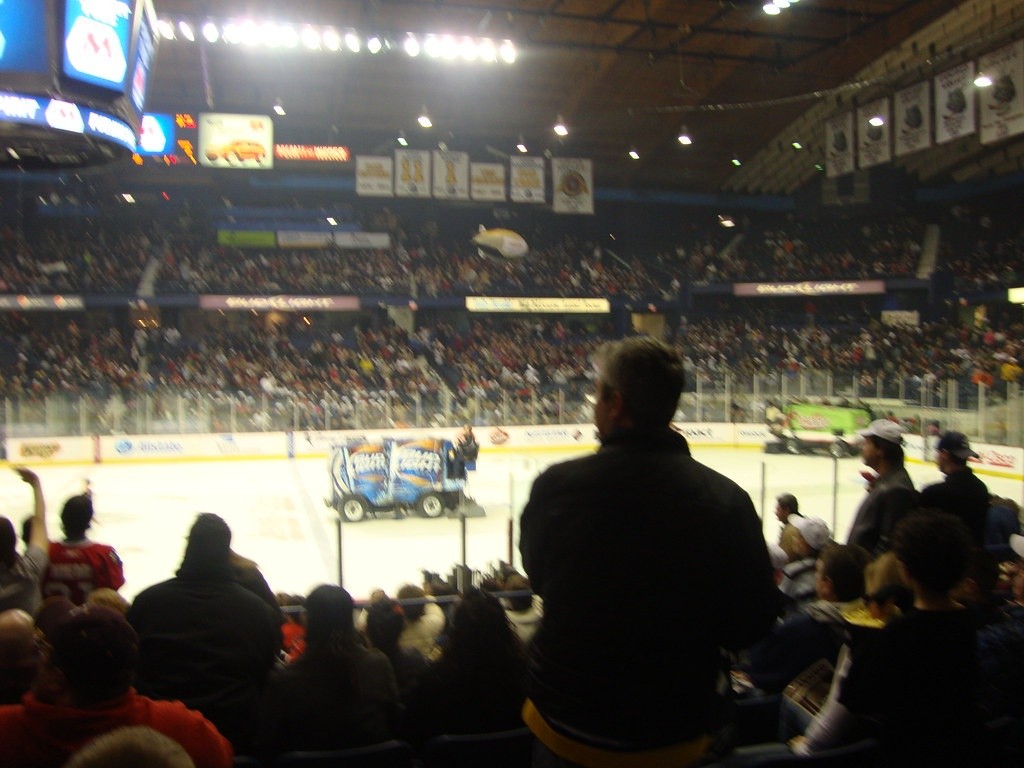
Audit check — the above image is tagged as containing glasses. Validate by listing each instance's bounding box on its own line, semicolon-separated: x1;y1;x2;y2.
860;588;893;607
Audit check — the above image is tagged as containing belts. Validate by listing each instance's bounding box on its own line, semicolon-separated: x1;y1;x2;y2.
523;699;707;768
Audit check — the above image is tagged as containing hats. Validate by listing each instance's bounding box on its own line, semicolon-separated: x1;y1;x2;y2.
778;495;802;517
1008;533;1024;557
861;419;903;443
788;512;829;550
939;433;981;460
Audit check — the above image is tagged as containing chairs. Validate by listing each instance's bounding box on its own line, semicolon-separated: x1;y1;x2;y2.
738;740;880;768
285;738;410;768
735;695;782;746
421;727;536;768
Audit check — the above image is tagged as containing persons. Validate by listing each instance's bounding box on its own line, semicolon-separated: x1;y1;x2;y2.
518;337;772;768
501;573;543;640
0;194;581;428
0;461;535;768
581;210;1024;411
454;422;478;464
775;410;1024;768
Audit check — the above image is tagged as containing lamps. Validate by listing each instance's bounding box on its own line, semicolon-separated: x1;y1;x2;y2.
516;134;529;153
678;124;693;145
973;72;992;87
720;215;736;228
417;103;434;129
553;112;570;136
868;114;884;126
273;97;286;115
